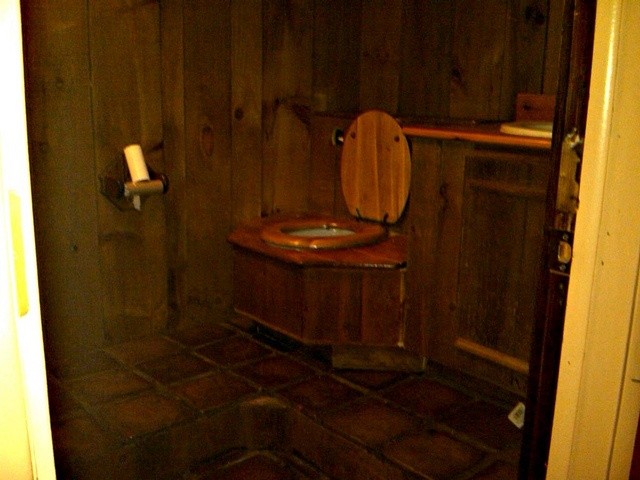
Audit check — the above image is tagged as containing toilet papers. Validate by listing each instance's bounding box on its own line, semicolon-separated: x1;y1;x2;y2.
122;144;151;184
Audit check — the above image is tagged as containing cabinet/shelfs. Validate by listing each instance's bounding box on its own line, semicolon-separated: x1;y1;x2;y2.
407;139;550;369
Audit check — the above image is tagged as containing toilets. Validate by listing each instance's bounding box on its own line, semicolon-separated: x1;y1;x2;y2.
226;109;411;346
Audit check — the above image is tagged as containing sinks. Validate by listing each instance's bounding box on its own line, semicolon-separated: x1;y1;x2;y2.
520;121;554;132
